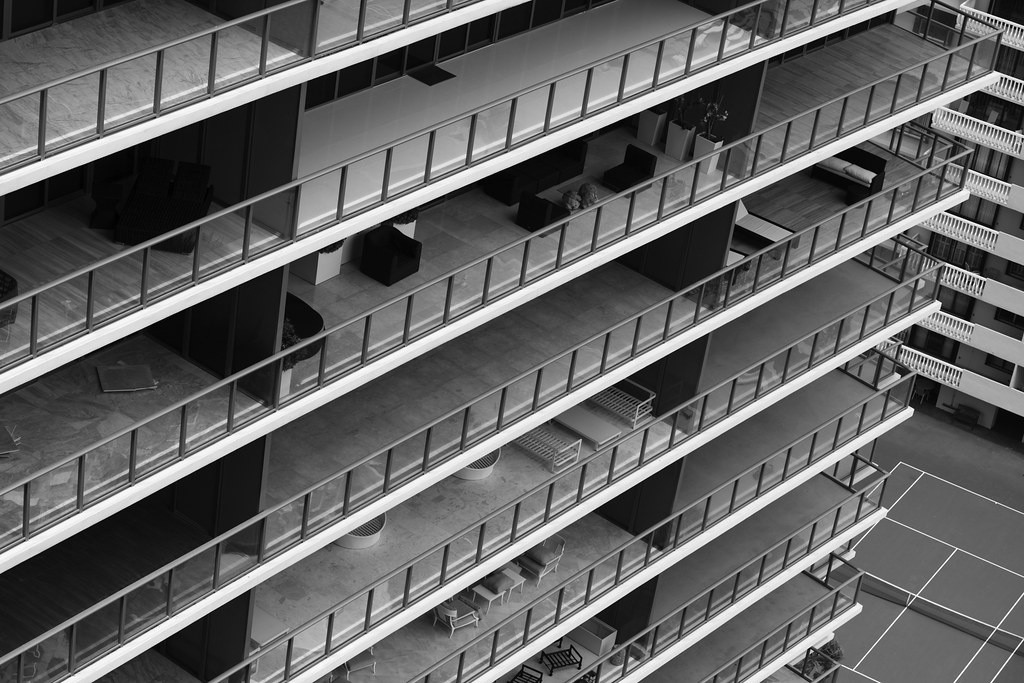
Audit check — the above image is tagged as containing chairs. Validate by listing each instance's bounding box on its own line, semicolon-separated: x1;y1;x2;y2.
342;646;376;680
432;601;479;639
911;382;929;405
516;534;566;588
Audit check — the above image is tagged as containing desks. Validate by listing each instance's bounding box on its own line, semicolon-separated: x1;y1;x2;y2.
915;380;935;398
548;404;622;452
471;568;526;616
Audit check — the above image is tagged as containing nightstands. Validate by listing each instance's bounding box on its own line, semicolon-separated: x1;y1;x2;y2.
88;183;123;231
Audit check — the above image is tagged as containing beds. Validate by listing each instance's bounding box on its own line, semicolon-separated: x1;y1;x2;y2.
733;199;801;261
114;156;214;256
724;247;751;286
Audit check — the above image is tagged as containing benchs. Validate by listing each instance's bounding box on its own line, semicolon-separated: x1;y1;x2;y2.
589;378;656;430
513;425;583;475
950;403;980;431
809;146;888;196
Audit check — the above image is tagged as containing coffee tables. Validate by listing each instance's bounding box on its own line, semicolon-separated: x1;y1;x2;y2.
556;175;619;212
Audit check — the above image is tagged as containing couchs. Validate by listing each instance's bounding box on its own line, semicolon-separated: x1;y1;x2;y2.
359;225;423;287
483;138;588;207
602;144;657;198
515;190;571;238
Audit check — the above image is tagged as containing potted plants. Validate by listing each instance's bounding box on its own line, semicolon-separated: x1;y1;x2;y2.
637;92;729;175
279;317;308;399
